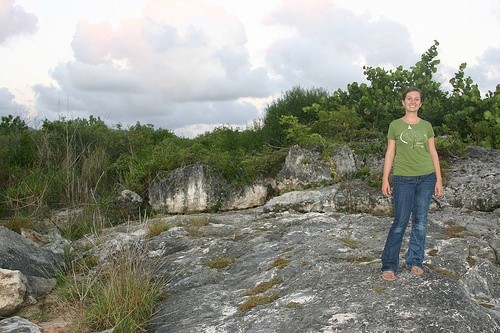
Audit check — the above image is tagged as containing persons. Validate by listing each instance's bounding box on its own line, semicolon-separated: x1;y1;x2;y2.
380;86;443;282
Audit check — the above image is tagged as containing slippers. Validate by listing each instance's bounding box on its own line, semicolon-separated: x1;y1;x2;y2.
406;263;424;275
382;270;395;280
